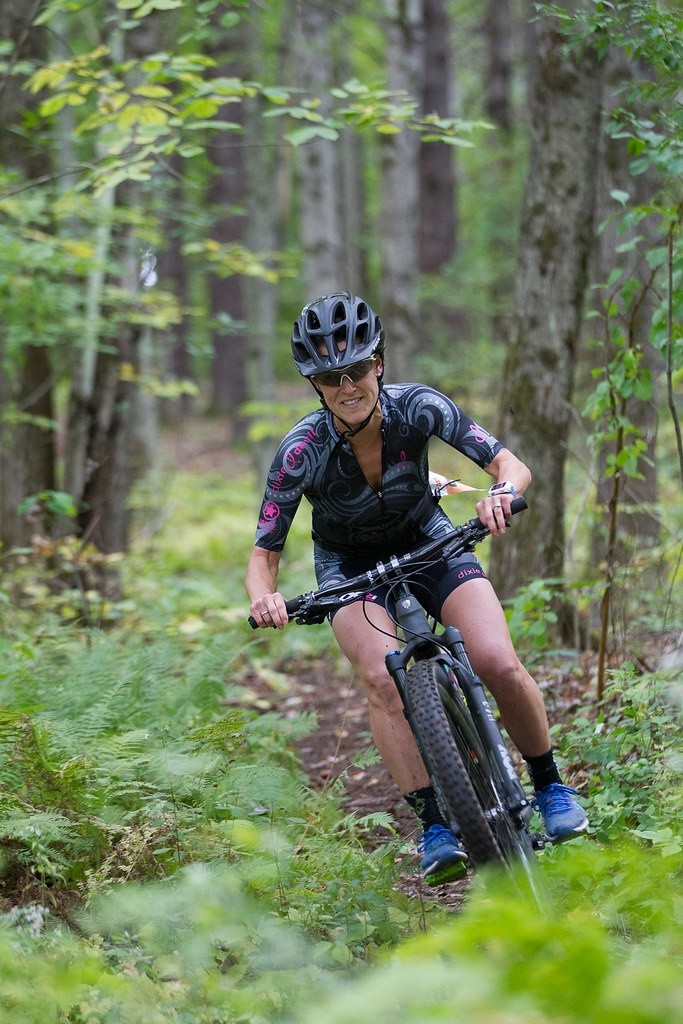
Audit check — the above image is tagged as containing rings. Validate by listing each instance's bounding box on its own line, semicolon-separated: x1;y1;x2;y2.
261;610;269;616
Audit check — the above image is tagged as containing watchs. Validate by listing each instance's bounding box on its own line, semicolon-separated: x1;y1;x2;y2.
488;481;517;498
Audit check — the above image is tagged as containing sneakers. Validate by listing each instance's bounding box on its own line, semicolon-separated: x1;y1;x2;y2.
531;781;590;842
416;824;468;879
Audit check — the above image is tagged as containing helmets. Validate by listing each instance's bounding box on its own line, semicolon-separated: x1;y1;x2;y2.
290;290;386;376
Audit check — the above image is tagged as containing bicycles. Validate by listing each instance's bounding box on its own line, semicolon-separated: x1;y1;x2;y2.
252;493;558;933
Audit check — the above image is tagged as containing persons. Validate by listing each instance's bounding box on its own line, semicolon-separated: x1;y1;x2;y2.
245;289;589;879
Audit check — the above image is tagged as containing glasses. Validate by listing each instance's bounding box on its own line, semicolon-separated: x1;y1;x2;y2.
311;353;378;387
